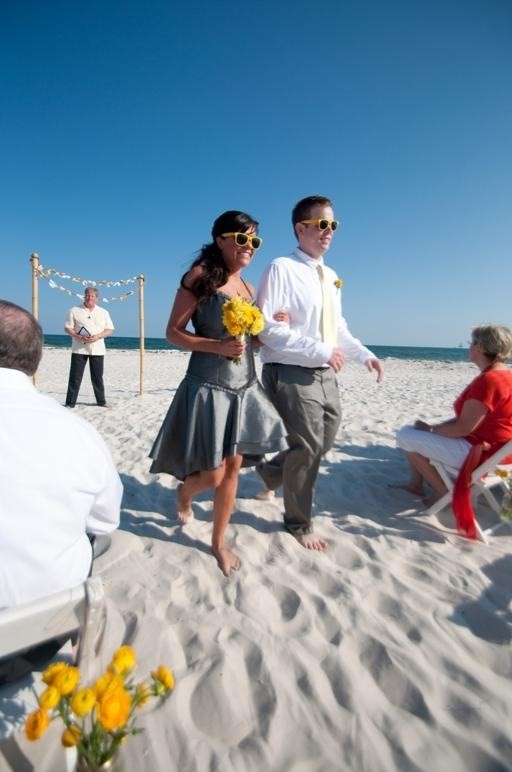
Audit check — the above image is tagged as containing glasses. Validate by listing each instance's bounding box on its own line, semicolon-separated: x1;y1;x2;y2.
220;231;263;250
301;217;340;232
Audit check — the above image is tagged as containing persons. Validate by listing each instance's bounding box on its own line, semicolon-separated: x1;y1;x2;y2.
254;195;383;550
385;319;512;508
147;208;288;578
62;287;116;408
1;301;126;686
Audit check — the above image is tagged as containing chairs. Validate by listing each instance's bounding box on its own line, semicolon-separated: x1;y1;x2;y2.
0;576;105;772
422;438;512;543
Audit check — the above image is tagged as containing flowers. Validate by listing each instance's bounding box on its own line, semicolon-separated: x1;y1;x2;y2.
334;279;343;292
223;297;264;364
25;647;176;772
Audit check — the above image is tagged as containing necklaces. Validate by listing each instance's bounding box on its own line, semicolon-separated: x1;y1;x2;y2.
228;280;241;296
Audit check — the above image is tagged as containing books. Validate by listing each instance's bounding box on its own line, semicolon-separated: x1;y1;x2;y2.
77;326;91;345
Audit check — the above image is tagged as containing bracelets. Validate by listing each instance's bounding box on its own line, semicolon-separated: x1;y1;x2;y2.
430;425;435;433
